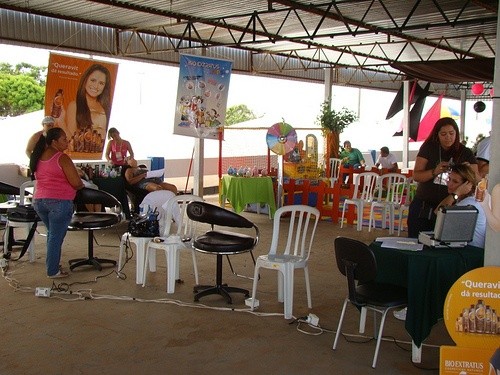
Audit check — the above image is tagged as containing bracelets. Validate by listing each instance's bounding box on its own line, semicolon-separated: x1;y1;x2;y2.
432;169;438;180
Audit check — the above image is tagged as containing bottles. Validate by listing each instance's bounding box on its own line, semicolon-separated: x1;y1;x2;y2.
91;130;97;152
78;129;84;152
51;89;63;118
455;318;458;331
475;300;485;333
458;313;463;331
100;164;122;179
485;305;491;334
463;309;469;332
95;165;99;178
74;131;78;152
227;165;277;177
469;305;475;332
97;134;101;152
76;163;93;180
491;309;496;334
68;136;74;152
496;316;500;334
84;127;91;152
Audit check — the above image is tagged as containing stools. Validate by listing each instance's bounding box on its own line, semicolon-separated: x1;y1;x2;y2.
69;187;120;271
0;180;28;255
186;201;260;305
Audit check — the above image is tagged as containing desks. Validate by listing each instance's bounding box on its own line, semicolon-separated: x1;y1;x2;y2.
221;175;277;217
370;239;482;364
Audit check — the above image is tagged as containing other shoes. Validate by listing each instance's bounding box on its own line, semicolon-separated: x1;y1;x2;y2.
47;264;71;279
393;307;407;320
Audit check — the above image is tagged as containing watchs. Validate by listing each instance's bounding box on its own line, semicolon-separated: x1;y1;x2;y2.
449;193;459;203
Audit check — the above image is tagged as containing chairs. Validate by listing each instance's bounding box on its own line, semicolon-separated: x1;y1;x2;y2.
140;195;203;293
332;236;408;369
117;190;179;284
250;205;320;320
328;158;414;235
2;180;45;262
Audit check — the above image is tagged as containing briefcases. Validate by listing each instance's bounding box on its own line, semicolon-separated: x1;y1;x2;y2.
418;205;479;248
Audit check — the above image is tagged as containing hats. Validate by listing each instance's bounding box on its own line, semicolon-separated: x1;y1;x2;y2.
43;116;55;124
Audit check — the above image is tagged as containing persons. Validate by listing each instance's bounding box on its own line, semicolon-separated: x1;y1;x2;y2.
124;156;177;195
29;127;85;278
412;117;474;196
475;182;500;234
51;64;111;153
375;147;397;169
106;128;134;166
76;167;101;212
338;141;366;183
25;116;55;159
434;136;491;248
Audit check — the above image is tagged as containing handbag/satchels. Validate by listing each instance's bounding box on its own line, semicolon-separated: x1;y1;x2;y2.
7;203;41;223
130;210;160;237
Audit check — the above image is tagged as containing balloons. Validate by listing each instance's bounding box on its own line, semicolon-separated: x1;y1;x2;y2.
474;102;486;112
471;83;484;95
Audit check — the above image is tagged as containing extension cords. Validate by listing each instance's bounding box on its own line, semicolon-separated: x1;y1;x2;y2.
308;313;319;326
51;289;72;295
